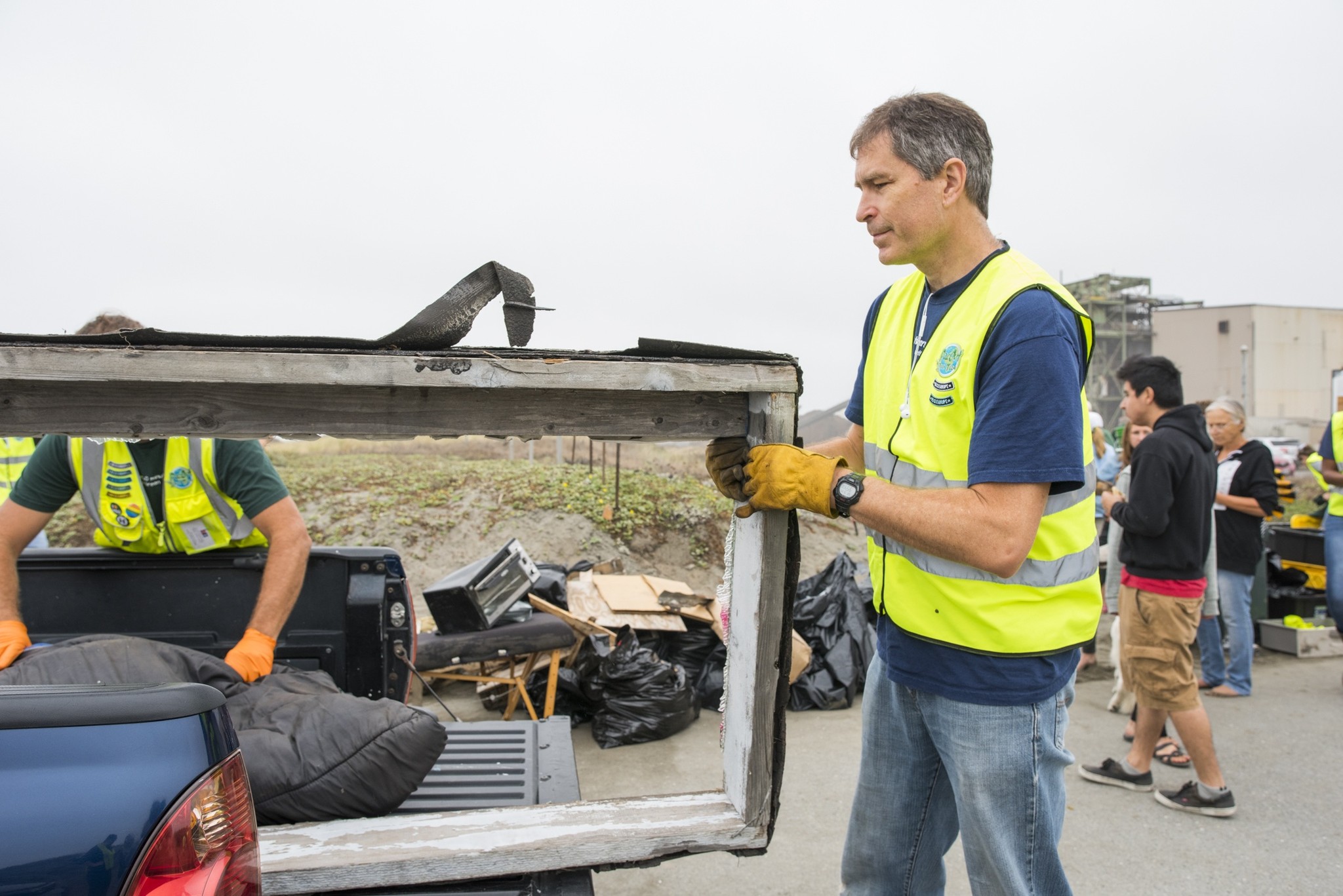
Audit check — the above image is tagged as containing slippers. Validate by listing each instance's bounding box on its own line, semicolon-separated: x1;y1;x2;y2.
1153;740;1191;768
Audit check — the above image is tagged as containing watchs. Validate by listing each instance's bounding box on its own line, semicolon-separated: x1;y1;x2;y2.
832;470;866;518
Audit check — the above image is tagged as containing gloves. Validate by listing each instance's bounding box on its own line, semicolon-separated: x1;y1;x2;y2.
733;444;850;518
702;435;748;501
225;627;277;684
1;620;31;669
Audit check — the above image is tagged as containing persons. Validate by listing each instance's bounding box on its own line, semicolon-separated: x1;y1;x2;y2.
1075;351;1241;818
702;91;1102;895
1297;441;1335;517
1;315;316;686
1320;410;1343;635
1079;409;1193;769
1;436;50;548
1181;393;1276;694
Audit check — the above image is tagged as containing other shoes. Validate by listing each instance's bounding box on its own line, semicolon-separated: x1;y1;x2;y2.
1204;688;1250;697
1197;676;1214;688
1075;657;1099;675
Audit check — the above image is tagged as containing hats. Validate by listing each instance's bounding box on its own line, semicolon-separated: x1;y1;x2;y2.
1089;411;1104;430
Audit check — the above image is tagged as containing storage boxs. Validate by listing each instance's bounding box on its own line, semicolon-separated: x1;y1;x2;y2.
1257;615;1336;653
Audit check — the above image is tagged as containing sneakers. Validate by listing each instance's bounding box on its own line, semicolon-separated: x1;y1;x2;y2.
1077;757;1154;791
1154;780;1237;817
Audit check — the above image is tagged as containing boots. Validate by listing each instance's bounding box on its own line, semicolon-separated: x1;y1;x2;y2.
1123;727;1173;742
